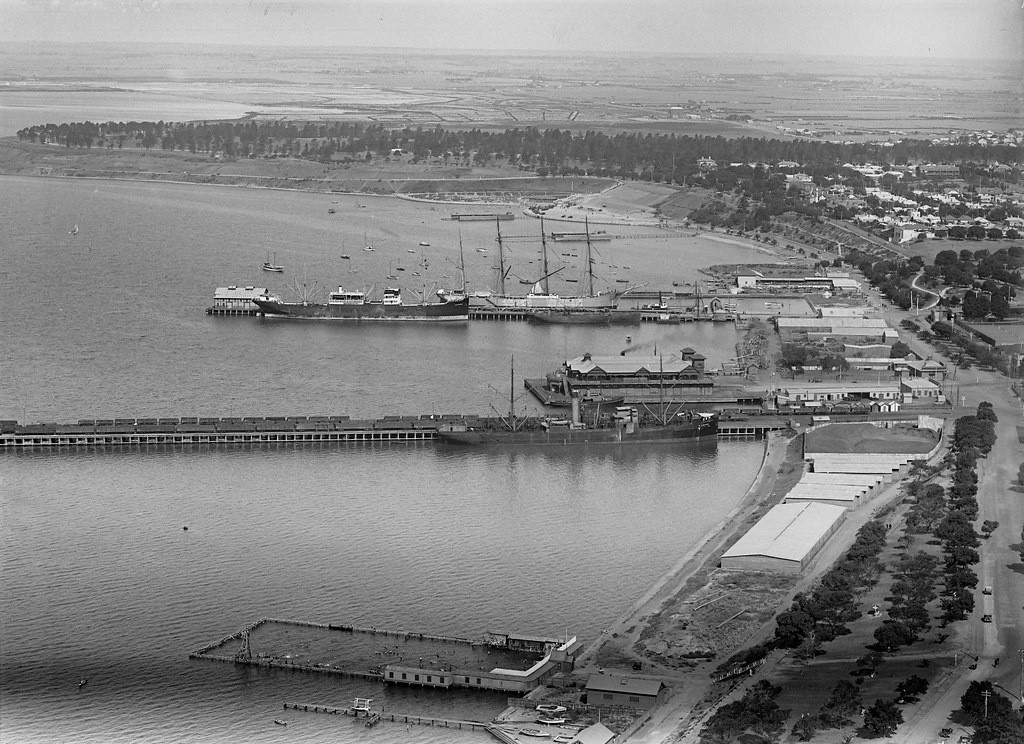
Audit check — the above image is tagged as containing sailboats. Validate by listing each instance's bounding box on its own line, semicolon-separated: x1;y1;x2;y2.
262;251;284;272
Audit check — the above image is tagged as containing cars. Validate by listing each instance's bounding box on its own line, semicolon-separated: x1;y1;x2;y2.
984;586;993;594
984;614;993;622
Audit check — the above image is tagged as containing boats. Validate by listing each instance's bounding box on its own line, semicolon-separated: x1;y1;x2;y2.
553;735;574;742
535;704;567;713
536;716;566;723
434;352;719;445
526;309;613;325
520;727;550;737
252;294;469;321
